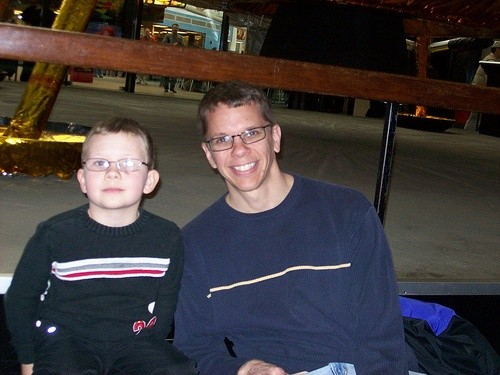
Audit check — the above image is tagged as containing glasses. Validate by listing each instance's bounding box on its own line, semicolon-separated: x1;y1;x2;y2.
83;158;149;171
206;125;271;152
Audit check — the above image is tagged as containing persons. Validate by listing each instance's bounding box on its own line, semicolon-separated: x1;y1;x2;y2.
5;117;201;375
142;28;151;39
172;81;421;374
162;24;184;93
472;41;500;87
